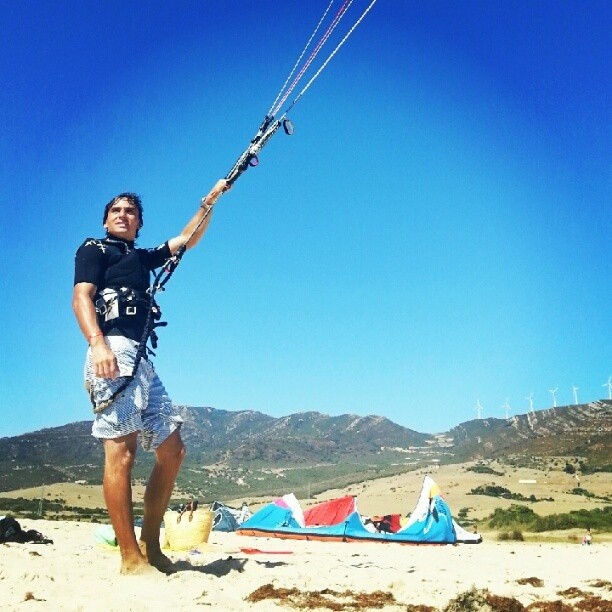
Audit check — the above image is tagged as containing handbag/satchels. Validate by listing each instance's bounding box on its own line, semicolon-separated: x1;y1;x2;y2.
163;501;214;550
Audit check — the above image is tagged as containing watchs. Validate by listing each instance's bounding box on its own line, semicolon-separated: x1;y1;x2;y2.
199;196;212;210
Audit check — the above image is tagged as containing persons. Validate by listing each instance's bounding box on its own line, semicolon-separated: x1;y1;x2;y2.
71;179;232;578
